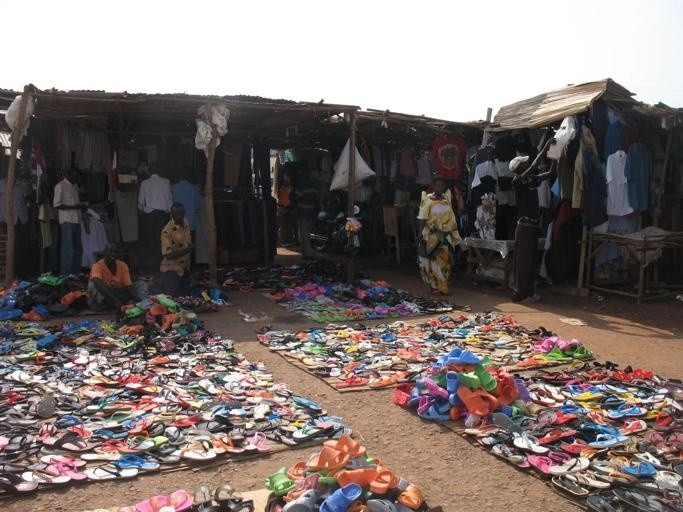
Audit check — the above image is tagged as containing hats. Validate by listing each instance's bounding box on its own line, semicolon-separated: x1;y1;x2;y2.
509;156;529;172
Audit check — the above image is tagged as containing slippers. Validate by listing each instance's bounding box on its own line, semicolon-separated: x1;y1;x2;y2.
424;295;683;511
0;266;423;512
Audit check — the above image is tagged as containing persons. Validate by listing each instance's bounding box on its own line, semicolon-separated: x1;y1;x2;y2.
53;166;90;272
138;162;173;259
417;175;462;296
292;165;321;249
171;169;204;271
159;202;194;287
0;163;33;282
87;241;148;309
